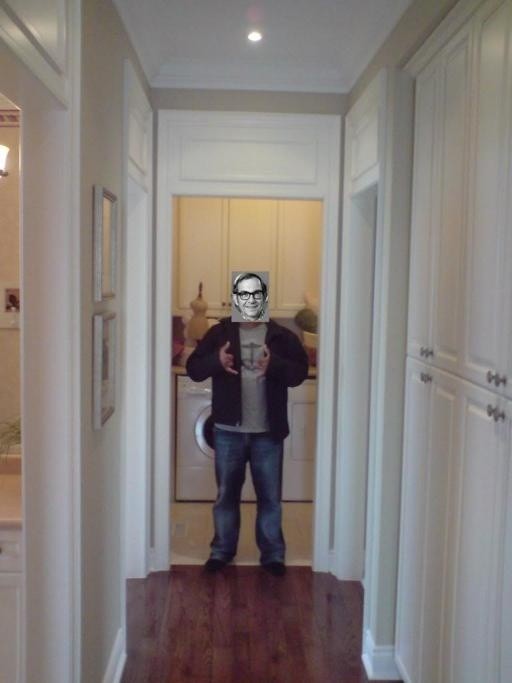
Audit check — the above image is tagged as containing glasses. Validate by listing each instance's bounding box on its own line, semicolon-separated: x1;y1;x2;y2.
235;291;263;300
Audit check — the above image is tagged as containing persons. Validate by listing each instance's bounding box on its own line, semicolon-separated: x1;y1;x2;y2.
232;269;270;321
185;316;311;578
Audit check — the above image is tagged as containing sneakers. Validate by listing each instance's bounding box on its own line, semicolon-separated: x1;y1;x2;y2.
264;562;285;576
205;559;226;572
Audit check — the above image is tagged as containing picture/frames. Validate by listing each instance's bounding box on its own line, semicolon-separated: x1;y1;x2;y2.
92;182;121;428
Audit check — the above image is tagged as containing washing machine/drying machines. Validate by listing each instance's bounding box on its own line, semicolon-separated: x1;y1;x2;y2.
281;379;317;501
174;376;257;502
